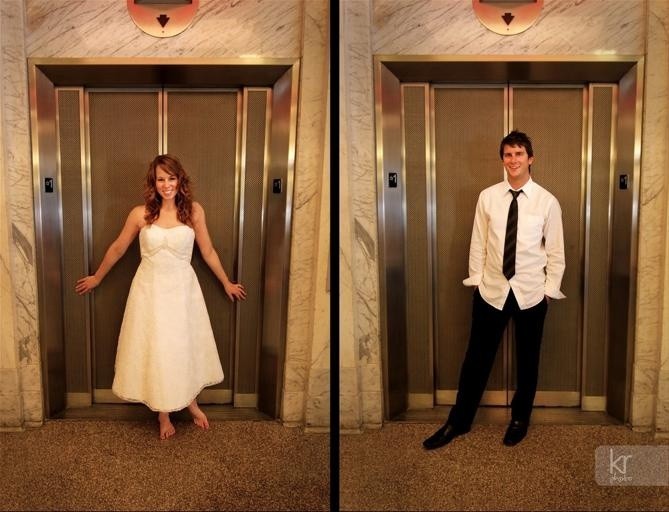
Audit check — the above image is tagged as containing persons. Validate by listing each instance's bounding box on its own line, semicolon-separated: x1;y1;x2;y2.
75;155;247;440
423;128;566;450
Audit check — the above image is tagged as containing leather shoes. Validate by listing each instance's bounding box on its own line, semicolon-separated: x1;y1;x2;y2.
503;417;529;446
423;418;472;450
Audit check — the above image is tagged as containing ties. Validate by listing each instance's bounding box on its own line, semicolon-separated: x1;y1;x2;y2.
503;189;523;281
